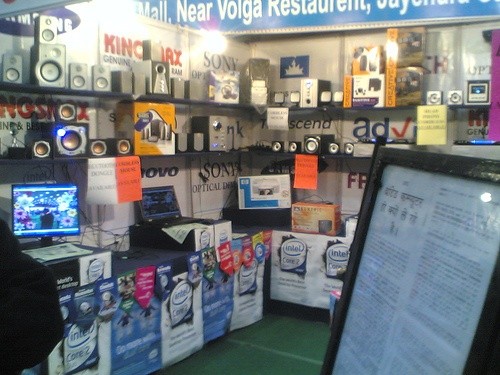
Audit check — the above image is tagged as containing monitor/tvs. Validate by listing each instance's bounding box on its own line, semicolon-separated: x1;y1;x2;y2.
11;183;81;248
321;145;500;375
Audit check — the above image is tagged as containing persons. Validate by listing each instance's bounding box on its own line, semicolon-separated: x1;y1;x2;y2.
40;208;54;229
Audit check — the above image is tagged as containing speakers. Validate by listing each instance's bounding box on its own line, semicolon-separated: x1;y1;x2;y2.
26;102;133;157
275;93;284;104
176;133;188;152
344;142;355;155
1;54;22;84
426;90;443;105
288;140;303;153
271;140;284;151
30;14;204;100
303;133;340;154
322;91;331;102
334;91;344;102
192;133;203;150
448;90;463;105
292;92;300;102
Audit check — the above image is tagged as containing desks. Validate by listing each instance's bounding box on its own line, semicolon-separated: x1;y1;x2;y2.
22;214;359;375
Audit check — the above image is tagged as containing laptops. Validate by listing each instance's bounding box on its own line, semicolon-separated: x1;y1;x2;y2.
136;186;205;227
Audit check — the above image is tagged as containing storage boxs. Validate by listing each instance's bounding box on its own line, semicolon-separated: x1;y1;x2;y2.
291;201;342;236
113;99;175;157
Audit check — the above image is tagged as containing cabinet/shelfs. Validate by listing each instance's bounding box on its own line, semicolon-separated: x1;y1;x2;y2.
0;82;500;164
